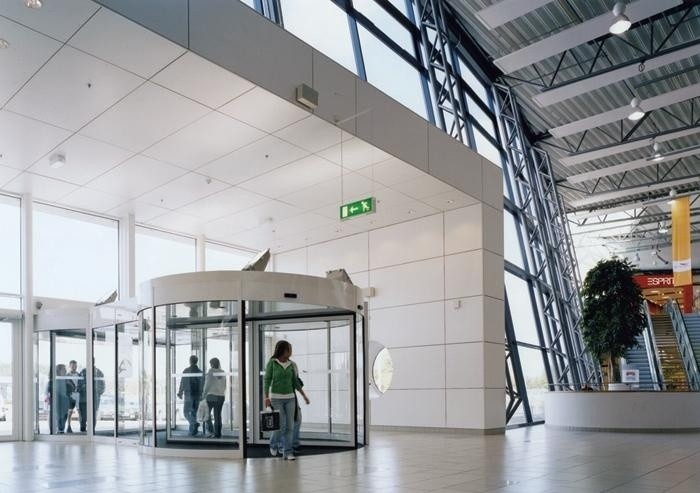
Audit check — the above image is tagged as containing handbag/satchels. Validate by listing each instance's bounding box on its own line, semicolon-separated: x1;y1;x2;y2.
259;405;280;431
196;398;209;423
68;397;76;409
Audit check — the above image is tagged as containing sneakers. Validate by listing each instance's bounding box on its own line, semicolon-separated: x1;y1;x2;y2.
55;425;73;434
270;447;277;456
283;453;295;460
205;432;221;439
278;446;284;455
80;424;86;431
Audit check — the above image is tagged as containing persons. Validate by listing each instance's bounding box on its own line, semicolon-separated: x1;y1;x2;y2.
77;357;104;432
177;355;205;436
278;342;305;455
201;357;226;438
65;360;83;433
48;364;76;435
263;341;310;461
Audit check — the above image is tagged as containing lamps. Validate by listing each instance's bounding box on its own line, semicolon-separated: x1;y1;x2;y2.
607;0;646;122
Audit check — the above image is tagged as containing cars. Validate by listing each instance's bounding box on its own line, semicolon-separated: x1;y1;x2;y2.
0;405;8;422
39;408;47;422
72;409;79;422
100;409;133;421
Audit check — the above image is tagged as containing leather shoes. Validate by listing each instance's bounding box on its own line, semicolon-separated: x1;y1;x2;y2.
188;423;200;436
